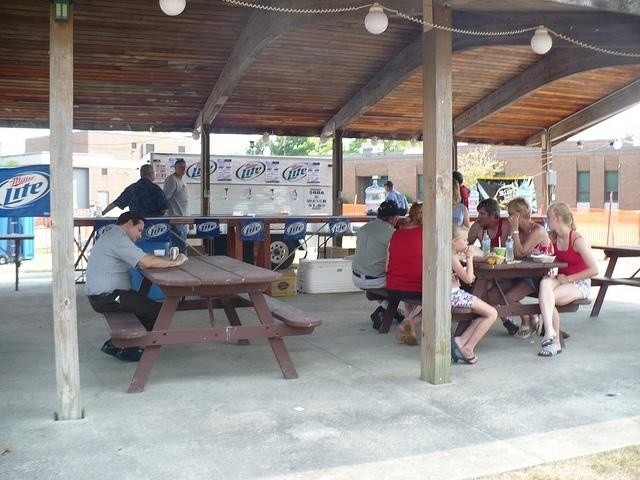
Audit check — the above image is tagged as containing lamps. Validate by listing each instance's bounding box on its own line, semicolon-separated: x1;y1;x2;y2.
158;0;187;17
53;0;71;21
530;24;552;55
364;3;388;35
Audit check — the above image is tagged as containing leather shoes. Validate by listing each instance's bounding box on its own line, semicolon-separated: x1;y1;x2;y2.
101;342;121;356
116;349;142;361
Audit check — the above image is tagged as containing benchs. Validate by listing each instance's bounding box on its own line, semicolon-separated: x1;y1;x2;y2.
234;292;321;338
528;293;591;312
591;277;640;287
103;298;151;348
362;288;472;321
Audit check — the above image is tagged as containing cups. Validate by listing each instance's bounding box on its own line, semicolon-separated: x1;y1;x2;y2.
493;246;507;257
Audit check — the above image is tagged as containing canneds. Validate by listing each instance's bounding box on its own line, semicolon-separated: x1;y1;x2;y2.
170;247;179;260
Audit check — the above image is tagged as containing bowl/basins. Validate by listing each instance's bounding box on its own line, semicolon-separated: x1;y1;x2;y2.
533;255;556;263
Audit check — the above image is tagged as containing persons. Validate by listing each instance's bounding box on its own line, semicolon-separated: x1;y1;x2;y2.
352;181;423;346
85;210;190;361
162;159;195;254
452;173;601;367
98;164;168;217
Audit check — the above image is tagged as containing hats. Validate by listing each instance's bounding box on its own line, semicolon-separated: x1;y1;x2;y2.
378;200;406;216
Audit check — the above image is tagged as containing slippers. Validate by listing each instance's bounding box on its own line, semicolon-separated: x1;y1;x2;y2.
397;320;417;345
503;317;562;356
454;347;478;364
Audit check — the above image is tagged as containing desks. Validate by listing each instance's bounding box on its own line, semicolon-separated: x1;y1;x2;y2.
590;245;640;316
379;259;568;348
127;255;299;393
0;234;34;291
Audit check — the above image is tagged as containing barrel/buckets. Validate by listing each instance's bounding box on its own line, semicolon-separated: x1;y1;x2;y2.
365;175;385;211
468;186;480;215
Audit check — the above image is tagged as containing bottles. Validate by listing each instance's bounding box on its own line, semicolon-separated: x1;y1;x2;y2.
505;235;515;263
482;230;491;253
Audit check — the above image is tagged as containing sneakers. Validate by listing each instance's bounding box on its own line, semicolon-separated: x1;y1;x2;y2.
371;307;385;329
394;309;405;324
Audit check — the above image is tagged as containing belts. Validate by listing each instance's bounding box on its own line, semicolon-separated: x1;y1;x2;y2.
353;271;377;279
